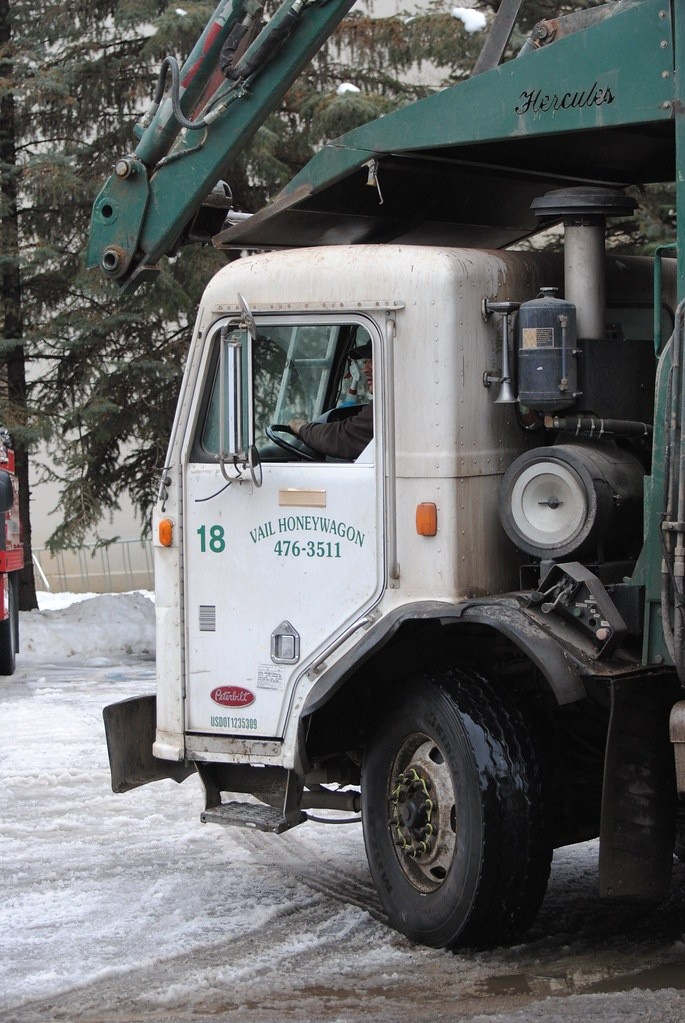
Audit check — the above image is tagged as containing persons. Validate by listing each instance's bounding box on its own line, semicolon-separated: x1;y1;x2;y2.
287;339;373;459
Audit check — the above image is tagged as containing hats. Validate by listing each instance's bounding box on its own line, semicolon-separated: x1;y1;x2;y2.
349;338;372;359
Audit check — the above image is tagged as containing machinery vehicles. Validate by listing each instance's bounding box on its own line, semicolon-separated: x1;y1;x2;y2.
84;6;684;949
